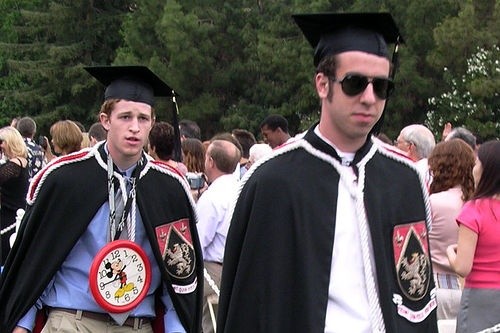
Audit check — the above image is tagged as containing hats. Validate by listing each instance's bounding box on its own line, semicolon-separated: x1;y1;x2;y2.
293;12;406;56
84;66;180;106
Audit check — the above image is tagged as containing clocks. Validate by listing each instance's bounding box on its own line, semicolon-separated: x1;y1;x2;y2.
91;242;151;313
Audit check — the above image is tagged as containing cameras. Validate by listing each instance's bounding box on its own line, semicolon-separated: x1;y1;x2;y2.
40;136;47;150
188;177;204;190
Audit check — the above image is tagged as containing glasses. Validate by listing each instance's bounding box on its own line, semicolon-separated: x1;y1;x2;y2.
394;140;416;145
328;76;395;99
